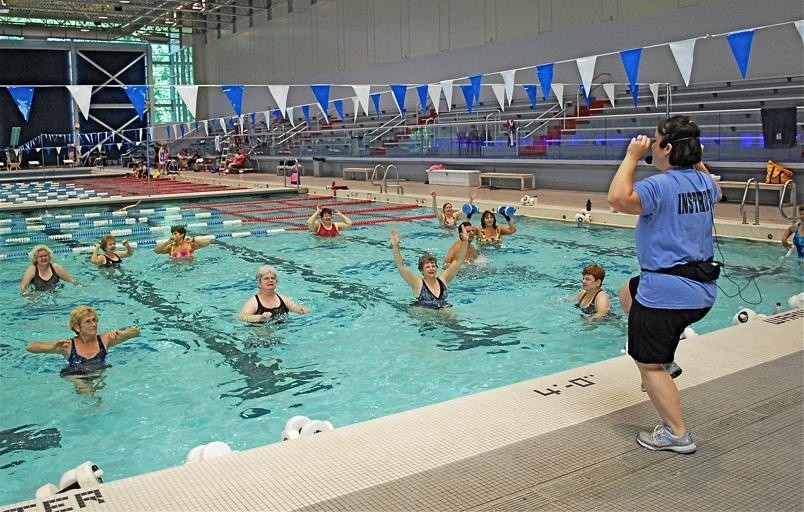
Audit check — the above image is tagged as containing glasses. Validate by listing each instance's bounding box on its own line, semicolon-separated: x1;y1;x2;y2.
86;318;98;325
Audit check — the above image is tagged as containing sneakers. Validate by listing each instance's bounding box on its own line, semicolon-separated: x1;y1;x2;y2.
640;360;682;393
636;420;696;455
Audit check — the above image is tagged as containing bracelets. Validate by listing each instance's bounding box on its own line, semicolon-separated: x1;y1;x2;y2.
193;237;194;242
315;214;318;216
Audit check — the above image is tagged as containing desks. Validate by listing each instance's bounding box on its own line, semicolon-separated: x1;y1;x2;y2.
424;169;482;188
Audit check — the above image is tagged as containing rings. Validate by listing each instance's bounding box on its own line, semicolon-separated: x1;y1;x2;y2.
637;138;642;141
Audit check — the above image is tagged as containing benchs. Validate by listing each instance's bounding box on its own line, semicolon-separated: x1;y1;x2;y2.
273;163;305;175
546;72;803;159
275;107;434;158
386;94;595;158
712;174;795;212
475;171;537;191
341;165;378;182
182;114;331;157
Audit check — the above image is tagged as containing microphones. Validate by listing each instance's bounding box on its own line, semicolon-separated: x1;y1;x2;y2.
645;155;652;164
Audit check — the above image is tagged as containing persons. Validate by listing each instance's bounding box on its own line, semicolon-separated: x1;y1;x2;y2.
444;222;478;268
467;124;478;142
118;200;141;212
390;224;469;311
574;264;623;324
430;191;477;229
152;224;210;259
238;264;310;324
607;116;724;455
465;209;517;248
432;108;437;120
90;235;135;269
93;142;246;179
332;181;337;190
781;205;804;260
19;244;83;297
307;203;353;238
481;124;494;145
26;305;140;376
507;118;516;149
